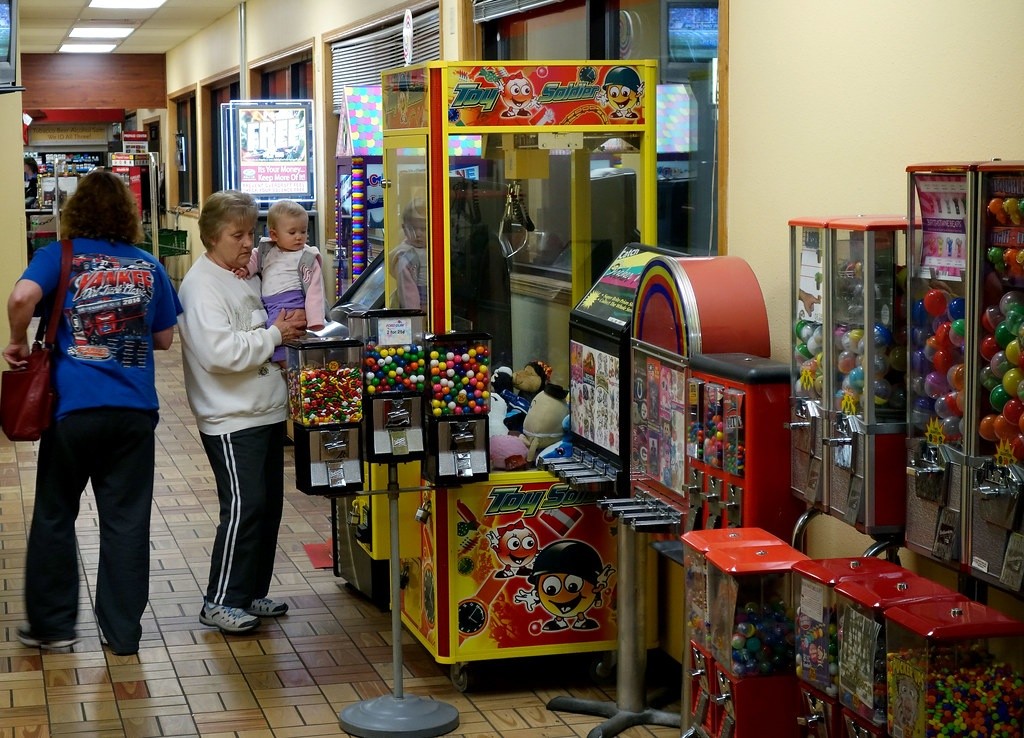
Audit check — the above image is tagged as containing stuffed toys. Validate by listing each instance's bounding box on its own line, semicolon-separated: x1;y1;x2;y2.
487;360;572;472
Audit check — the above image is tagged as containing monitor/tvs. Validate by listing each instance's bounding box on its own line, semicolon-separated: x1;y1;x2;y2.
325;250;385;321
0;0;16;82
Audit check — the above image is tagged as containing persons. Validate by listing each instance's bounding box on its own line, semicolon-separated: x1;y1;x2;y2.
24;154;39;261
177;190;307;633
231;200;325;368
2;171;185;657
387;197;432;313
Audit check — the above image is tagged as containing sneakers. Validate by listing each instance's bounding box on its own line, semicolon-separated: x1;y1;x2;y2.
244;596;288;617
199;596;262;636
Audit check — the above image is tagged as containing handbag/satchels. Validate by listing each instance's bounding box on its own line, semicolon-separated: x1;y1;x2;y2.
0;237;72;442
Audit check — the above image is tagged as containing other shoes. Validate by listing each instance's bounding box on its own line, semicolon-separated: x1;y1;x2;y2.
16;624;80;647
